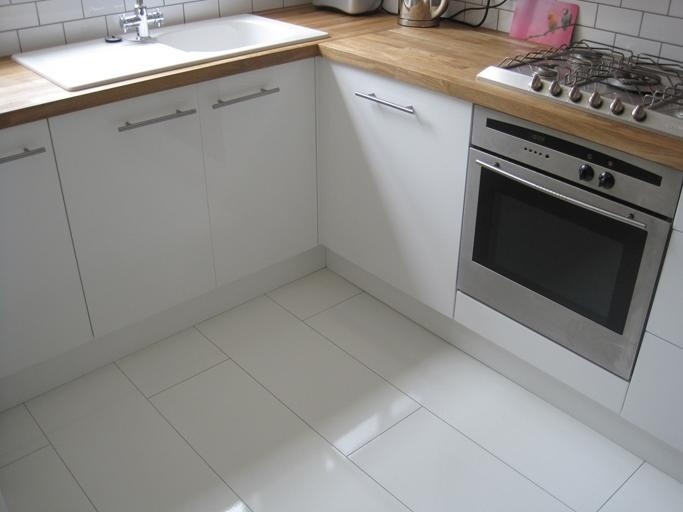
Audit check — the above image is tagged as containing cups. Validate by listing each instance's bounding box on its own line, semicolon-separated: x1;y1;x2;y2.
393;1;452;28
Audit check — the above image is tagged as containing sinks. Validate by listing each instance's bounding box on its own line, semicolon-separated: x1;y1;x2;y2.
159;14;328;54
11;37;186;91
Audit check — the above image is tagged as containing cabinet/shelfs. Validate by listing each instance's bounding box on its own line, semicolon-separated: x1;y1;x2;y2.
613;168;682;482
46;54;325;371
0;115;94;411
314;49;474;362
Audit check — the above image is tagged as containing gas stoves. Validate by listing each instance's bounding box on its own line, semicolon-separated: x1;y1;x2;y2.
476;37;682;140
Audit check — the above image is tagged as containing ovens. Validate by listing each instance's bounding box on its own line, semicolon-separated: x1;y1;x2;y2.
455;103;682;382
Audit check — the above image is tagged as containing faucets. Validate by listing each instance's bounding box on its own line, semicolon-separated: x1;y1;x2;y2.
119;5;162;37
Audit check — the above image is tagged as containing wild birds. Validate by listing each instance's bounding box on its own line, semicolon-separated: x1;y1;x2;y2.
561;8;572;31
547;10;558;34
